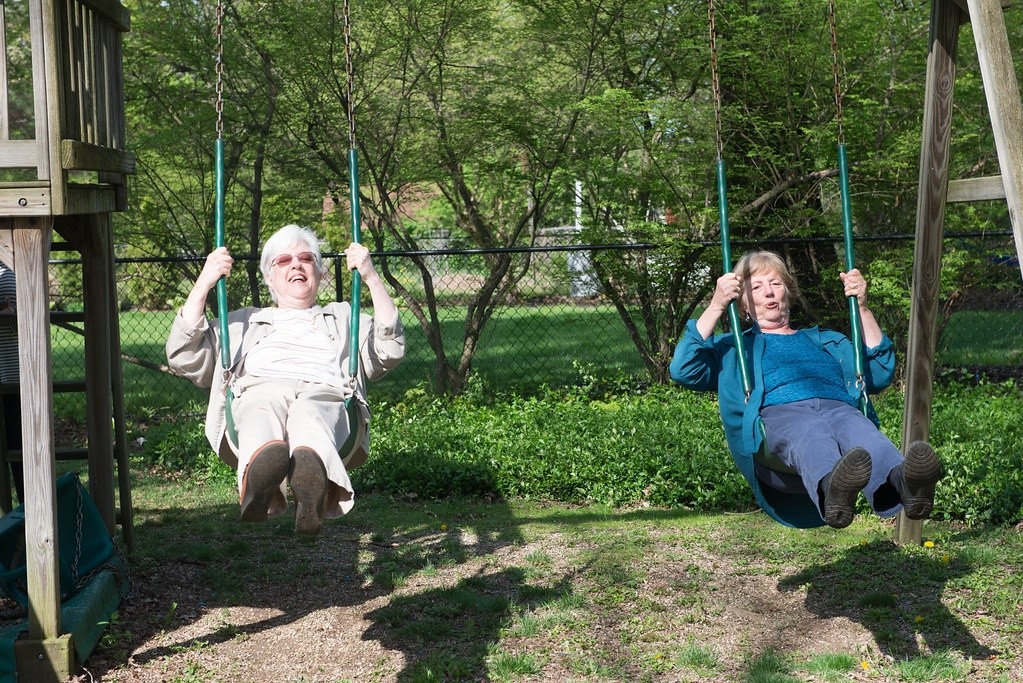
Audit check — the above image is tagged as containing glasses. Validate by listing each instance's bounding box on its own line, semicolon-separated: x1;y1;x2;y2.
268;252;320;270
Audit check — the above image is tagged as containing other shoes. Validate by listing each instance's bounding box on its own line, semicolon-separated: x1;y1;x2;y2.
238;440;289;523
287;447;330;537
823;446;873;529
898;440;938;519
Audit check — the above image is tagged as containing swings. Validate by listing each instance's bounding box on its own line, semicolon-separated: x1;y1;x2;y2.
211;1;364;486
699;1;878;529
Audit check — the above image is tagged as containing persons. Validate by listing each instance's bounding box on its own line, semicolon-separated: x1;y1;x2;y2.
165;225;405;538
0;259;25;513
668;251;940;529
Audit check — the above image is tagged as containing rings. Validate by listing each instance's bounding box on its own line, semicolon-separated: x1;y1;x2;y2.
854;283;858;289
